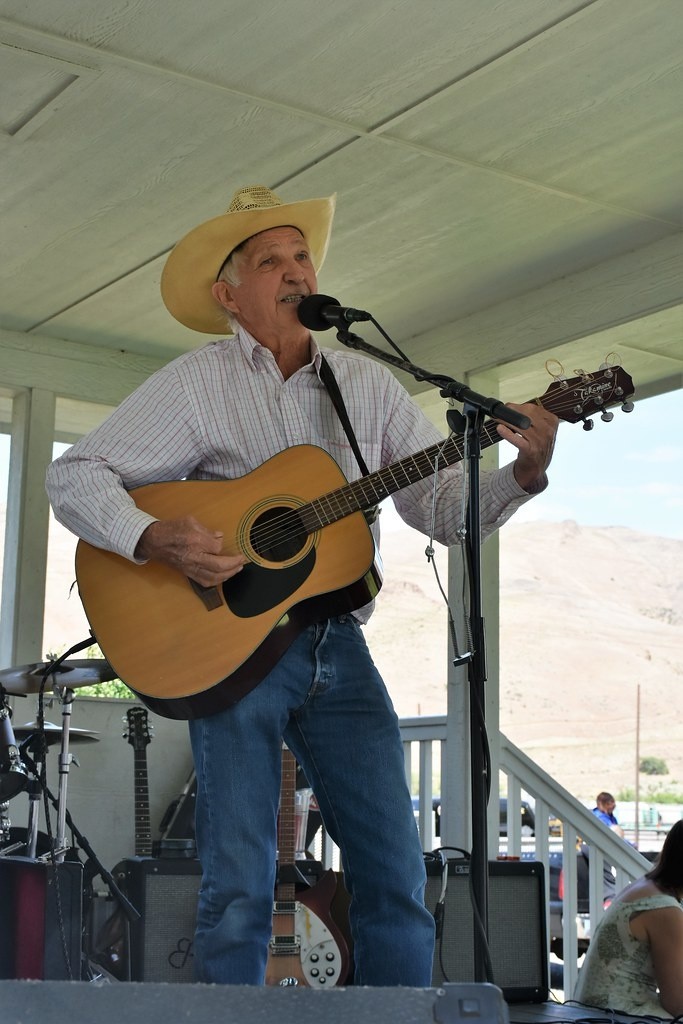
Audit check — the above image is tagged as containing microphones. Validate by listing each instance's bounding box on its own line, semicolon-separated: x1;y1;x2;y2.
297;293;373;331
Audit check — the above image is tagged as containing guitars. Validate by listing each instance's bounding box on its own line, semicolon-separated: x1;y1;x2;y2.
96;706;155;958
264;740;350;986
75;365;635;721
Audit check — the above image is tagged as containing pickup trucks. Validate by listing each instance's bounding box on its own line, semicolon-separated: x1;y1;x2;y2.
410;794;591;961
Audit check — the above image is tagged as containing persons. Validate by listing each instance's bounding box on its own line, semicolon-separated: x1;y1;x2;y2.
581;793;626;899
571;819;683;1018
47;184;560;989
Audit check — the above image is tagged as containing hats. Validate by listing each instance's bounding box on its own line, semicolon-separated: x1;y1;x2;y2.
160;186;337;336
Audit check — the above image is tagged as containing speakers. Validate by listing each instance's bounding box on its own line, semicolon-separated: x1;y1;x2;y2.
109;860;324;982
0;976;509;1024
421;858;549;1006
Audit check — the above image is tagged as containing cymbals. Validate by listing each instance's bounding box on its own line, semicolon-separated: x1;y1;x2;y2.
0;658;119;693
10;720;102;745
